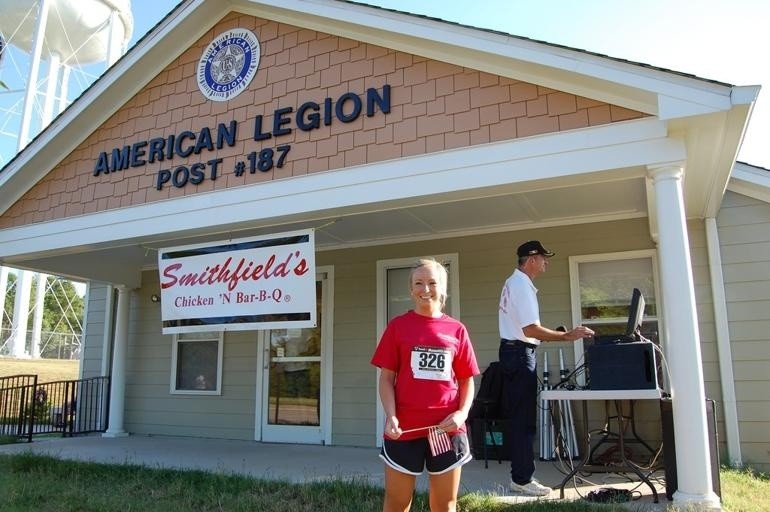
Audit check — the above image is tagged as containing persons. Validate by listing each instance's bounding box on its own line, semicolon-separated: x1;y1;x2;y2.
499;241;595;496
284;328;317;397
371;258;480;511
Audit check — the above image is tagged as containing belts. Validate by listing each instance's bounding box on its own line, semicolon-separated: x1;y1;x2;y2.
501;338;537;348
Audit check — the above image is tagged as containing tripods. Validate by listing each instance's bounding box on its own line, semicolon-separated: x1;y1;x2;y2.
557;350;581;460
471;398;502;469
538;351;556;461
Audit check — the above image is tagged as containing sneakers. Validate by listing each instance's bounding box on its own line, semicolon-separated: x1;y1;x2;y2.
509;474;553;496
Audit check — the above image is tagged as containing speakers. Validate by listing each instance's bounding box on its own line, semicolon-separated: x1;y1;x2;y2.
659;396;722;500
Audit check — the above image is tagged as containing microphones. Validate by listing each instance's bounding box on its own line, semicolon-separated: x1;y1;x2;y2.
555;325;567;332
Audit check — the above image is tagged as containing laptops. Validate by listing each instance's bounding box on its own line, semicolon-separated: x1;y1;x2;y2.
595;287;646;343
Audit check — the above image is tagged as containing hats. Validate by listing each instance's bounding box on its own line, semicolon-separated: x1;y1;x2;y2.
518;239;555;258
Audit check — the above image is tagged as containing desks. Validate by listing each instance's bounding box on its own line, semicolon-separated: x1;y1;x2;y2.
539;388;663;502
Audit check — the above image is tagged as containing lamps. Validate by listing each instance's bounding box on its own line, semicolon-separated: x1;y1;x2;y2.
151;294;160;303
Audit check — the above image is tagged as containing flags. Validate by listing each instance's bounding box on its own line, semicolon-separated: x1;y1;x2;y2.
427;426;453;457
